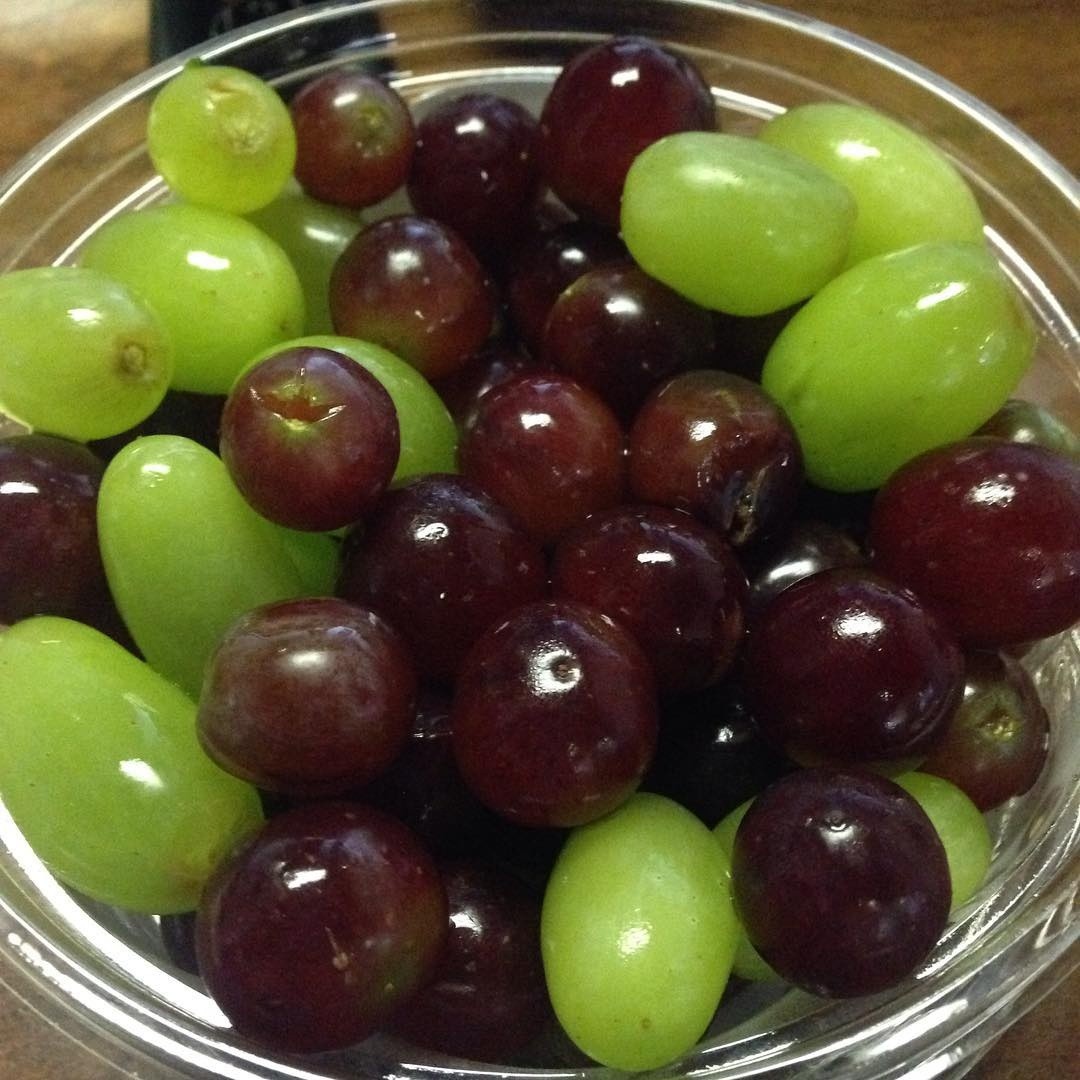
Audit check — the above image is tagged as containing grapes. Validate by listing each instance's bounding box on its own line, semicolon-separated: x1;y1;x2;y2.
1;34;1080;1079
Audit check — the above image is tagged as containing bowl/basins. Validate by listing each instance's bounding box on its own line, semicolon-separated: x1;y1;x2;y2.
1;0;1080;1079
